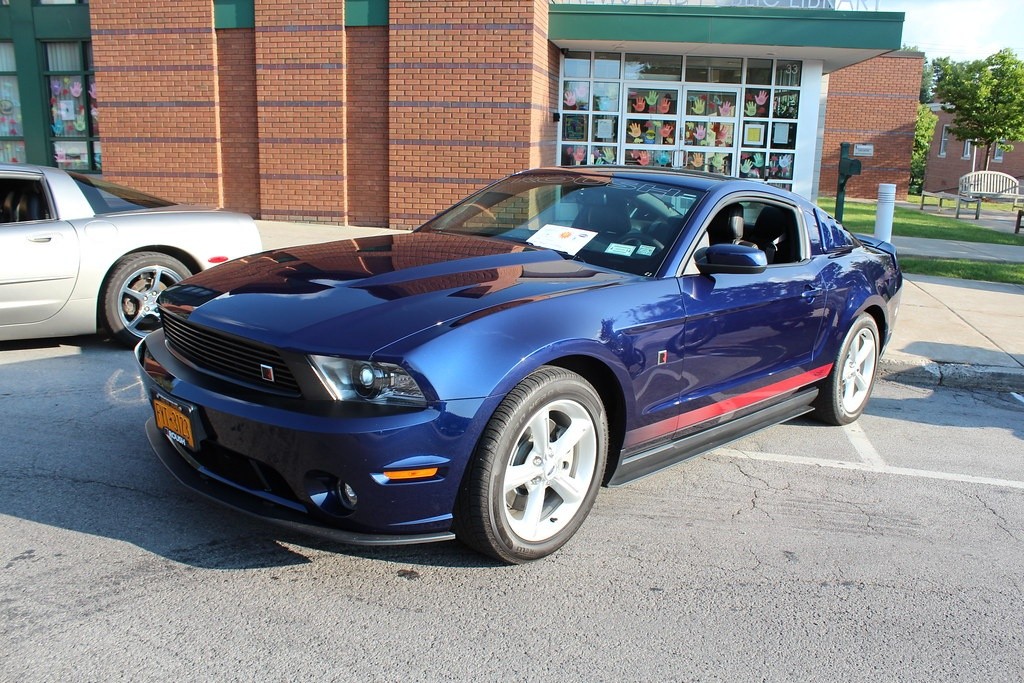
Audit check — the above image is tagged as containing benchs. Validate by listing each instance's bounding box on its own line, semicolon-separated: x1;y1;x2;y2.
920;190;981;219
958;170;1024;211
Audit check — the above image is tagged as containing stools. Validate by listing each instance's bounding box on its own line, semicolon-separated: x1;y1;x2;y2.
1014;210;1024;234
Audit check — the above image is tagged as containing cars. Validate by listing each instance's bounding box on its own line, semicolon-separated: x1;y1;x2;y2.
134;161;902;566
0;161;266;350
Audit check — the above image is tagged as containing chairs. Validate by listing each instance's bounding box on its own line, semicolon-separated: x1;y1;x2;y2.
706;203;759;249
585;197;642;246
